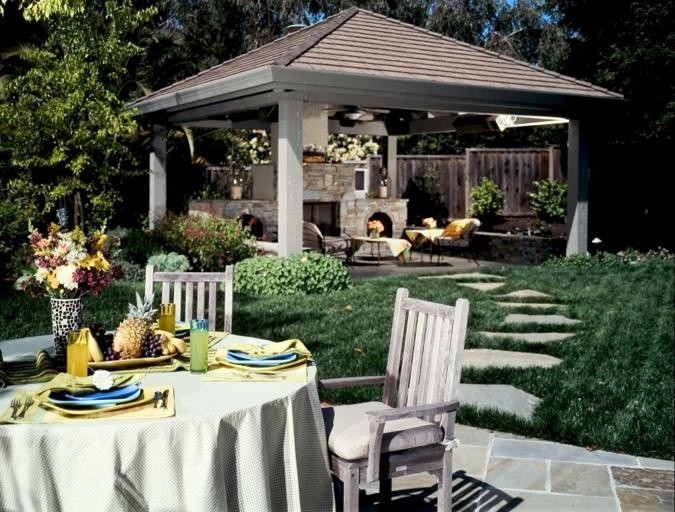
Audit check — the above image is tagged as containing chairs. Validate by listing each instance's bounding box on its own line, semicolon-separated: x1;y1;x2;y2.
437;218;480;266
316;287;470;512
144;263;233;333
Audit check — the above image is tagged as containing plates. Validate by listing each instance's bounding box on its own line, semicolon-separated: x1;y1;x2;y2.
228;350;294;360
32;390;158;415
88;353;179;368
226;354;297;366
65;385;139;400
48;390;142;406
218;358;308;371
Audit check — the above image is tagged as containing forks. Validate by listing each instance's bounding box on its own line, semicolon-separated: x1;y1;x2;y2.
17;396;33;419
10;399;21;420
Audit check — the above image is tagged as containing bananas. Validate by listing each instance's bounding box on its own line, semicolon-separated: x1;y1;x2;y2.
154;328;186;355
75;328;98;362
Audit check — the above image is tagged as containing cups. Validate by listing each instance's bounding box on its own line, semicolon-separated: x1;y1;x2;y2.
159;303;175;332
67;330;88;377
190;319;209;375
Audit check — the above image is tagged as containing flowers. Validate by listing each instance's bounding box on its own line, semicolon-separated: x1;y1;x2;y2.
368;220;384;232
13;216;122;300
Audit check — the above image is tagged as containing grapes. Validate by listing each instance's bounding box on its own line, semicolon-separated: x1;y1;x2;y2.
94;326;114;361
140;329;159;359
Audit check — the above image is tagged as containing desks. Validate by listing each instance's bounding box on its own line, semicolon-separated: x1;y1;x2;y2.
405;229;445;264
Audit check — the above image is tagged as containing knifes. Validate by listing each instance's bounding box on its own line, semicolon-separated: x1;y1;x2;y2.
161;390;170;408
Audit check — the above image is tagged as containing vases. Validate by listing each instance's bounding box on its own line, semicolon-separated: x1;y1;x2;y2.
49;298;81;359
370;229;379;238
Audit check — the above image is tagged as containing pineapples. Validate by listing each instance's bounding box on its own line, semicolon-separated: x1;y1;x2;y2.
114;291;155;359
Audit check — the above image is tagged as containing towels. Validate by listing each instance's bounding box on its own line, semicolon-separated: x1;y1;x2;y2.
225;335;312;358
35;371;145;396
